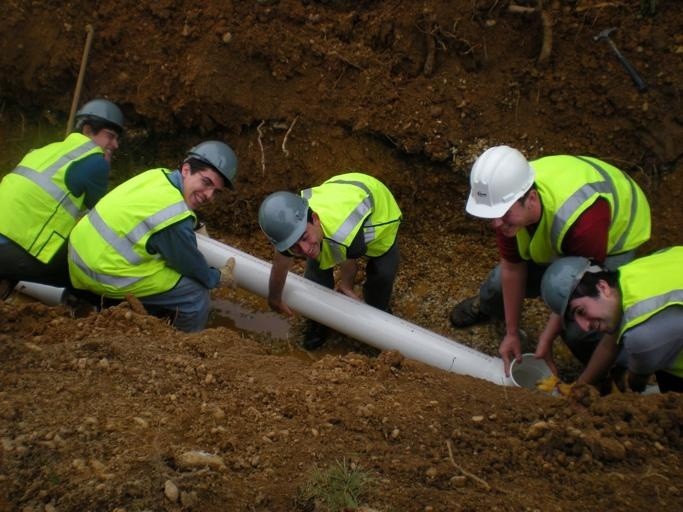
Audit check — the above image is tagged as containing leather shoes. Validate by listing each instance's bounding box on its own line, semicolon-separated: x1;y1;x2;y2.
449;296;484;329
304;318;327;350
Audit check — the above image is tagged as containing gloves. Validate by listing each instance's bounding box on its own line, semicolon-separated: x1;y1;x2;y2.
217;257;237;291
535;376;578;397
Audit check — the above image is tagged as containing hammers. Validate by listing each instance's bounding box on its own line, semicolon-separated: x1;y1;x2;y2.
593;27;648;92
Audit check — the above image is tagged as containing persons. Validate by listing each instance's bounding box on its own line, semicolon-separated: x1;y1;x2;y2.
259;173;403;359
66;140;238;334
0;100;127;311
541;245;683;397
450;146;651;378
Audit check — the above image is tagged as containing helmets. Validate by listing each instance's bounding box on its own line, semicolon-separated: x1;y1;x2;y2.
539;255;592;329
186;140;237;193
76;99;124;130
258;190;310;252
465;145;535;219
